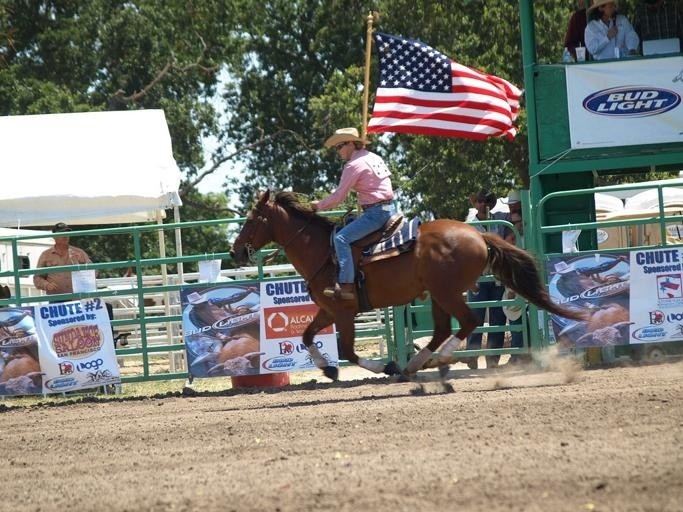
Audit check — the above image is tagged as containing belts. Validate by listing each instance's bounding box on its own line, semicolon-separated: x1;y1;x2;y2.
360;199;394;211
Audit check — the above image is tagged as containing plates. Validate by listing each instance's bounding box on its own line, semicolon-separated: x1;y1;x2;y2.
0;309;37;349
183;287;261;367
547;256;628;328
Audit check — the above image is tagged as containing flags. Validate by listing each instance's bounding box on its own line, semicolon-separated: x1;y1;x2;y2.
365;29;526;141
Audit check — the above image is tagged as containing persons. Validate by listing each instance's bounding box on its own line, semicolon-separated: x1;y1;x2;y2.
582;0;640;61
508;208;530;318
461;187;512;370
181;286;258;345
306;124;396;301
499;190;525;365
548;256;628;313
32;222;96;303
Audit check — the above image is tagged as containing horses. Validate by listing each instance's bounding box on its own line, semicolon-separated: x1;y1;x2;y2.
228;187;592;383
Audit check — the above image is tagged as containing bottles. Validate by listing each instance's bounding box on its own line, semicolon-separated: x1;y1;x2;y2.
548;320;557;345
563;46;571;63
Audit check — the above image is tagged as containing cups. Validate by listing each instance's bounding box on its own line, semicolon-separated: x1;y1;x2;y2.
198;260;222;282
575;46;586;63
72;269;97;295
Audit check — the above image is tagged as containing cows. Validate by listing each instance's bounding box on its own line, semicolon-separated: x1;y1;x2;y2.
576;305;636;346
207;336;266;374
0;354;47;396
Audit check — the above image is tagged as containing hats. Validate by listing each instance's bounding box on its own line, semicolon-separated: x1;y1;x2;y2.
501;289;528;321
469;188;497;211
500;192;522;205
321;127;372;149
52;223;73;233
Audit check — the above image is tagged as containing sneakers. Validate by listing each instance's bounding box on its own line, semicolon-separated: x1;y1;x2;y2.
486;358;500;369
466;358;478;369
498;358;523;370
323;284;356;300
